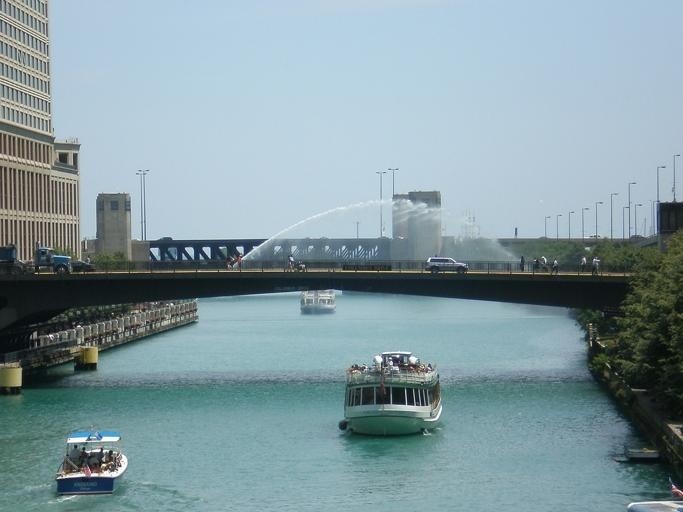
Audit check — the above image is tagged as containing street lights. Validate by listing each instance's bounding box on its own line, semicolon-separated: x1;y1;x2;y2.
377;168;386;239
135;168;150;240
655;165;665;201
671;153;680;200
388;166;398;196
542;182;643;238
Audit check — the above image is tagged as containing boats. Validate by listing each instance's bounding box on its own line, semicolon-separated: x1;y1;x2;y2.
338;348;444;438
297;290;336;314
54;429;128;497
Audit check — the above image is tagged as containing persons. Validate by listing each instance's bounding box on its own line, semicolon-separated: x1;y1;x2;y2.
70;444;113;468
349;355;433;374
519;256;560;273
287;254;295;271
591;257;601;275
226;254;242;271
580;255;587;273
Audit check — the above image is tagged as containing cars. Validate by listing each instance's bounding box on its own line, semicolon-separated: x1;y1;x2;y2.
70;260;97;273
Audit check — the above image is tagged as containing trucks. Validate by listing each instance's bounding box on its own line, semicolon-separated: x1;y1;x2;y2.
20;241;74;274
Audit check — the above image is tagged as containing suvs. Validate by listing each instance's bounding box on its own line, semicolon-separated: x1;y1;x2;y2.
424;256;470;274
